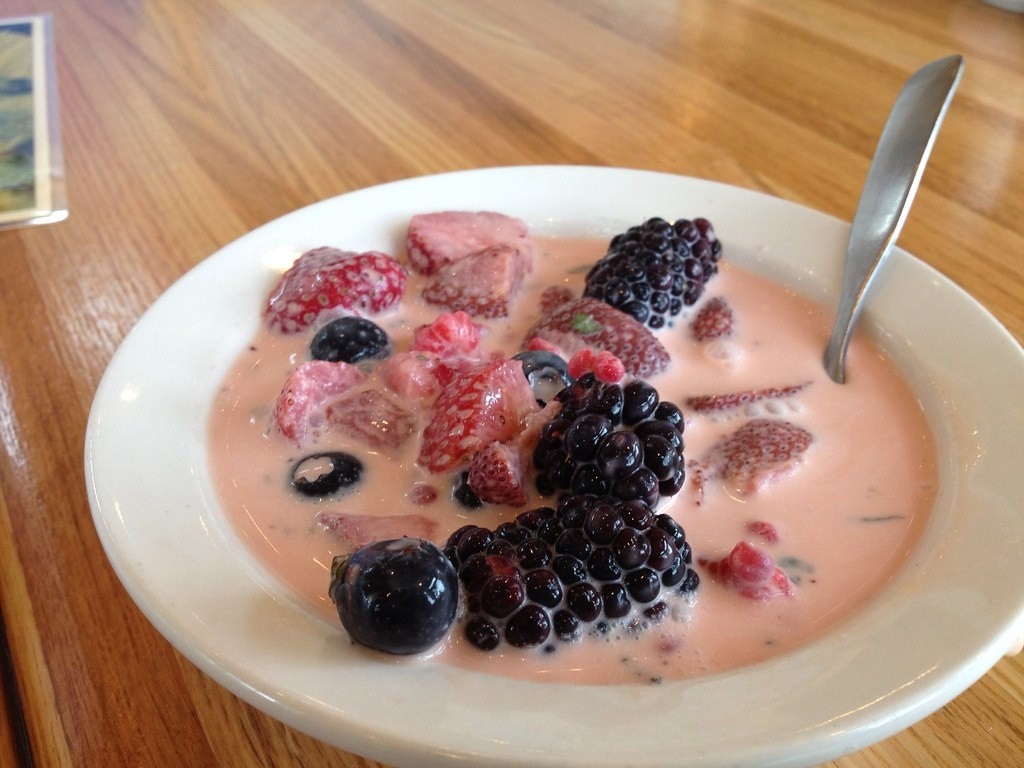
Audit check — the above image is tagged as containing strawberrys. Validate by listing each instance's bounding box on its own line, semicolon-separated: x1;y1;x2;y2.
263;210;818;607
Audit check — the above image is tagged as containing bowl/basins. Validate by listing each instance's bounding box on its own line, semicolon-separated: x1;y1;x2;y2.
84;164;1024;768
